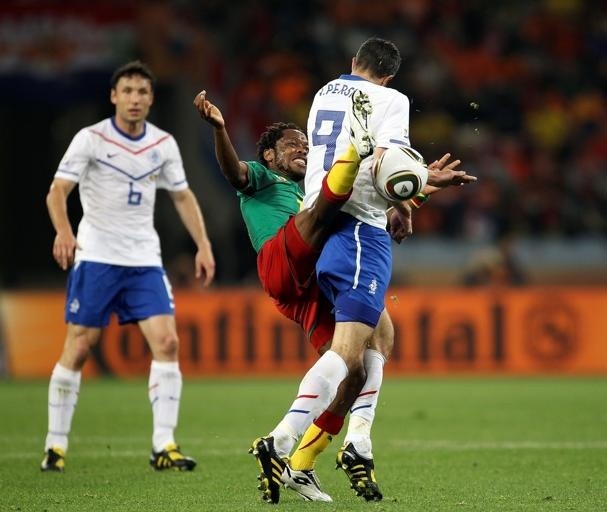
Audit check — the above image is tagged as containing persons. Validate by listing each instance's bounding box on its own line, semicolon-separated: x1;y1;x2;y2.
39;60;216;473
0;0;606;268
193;87;461;505
246;37;479;505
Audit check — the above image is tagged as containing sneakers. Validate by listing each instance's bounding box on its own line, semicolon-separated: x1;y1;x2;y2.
248;434;287;505
40;446;67;474
345;88;378;162
148;443;197;473
281;462;333;505
334;439;383;502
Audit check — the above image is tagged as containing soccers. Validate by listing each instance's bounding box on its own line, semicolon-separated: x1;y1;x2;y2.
371;147;427;202
